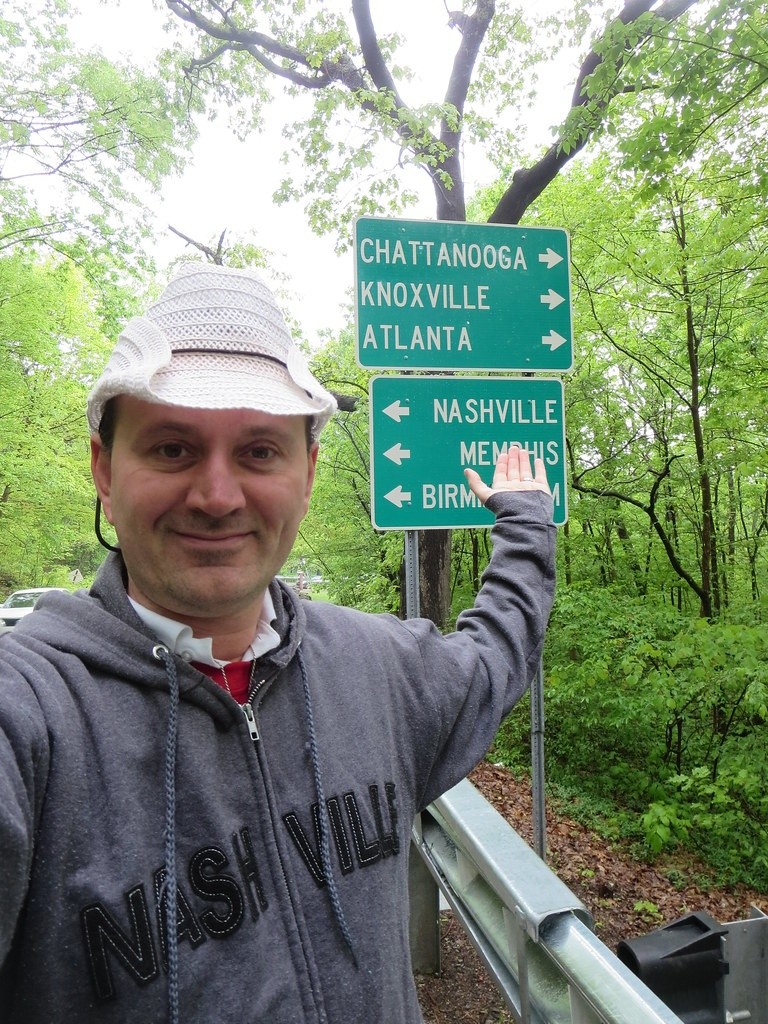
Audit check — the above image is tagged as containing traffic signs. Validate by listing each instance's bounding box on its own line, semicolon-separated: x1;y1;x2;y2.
355;222;575;373
373;378;571;529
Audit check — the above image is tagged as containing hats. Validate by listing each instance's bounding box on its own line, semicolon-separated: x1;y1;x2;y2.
87;262;339;450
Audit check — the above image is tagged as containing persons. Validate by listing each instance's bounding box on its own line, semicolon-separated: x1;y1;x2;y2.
0;266;560;1024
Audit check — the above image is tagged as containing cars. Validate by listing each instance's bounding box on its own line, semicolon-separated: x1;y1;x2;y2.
4;582;59;628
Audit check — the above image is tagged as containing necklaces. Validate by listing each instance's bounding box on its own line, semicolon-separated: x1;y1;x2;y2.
214;645;256;696
